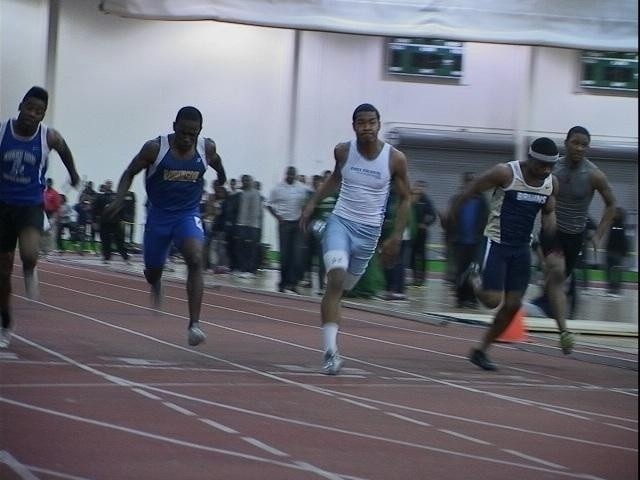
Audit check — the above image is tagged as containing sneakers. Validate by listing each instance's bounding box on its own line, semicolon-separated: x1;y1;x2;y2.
557;328;576;357
0;326;11;349
323;348;343;375
470;348;499;372
457;261;479;291
190;323;206;346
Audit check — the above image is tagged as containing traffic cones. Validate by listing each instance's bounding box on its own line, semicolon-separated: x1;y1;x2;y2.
496;283;539;344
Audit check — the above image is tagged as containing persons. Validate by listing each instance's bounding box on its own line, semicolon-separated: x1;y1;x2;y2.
575;204;627;297
0;85;81;351
43;167;339;296
355;172;488;308
534;211;597;319
451;137;558;375
540;126;617;355
102;104;227;347
299;103;412;376
